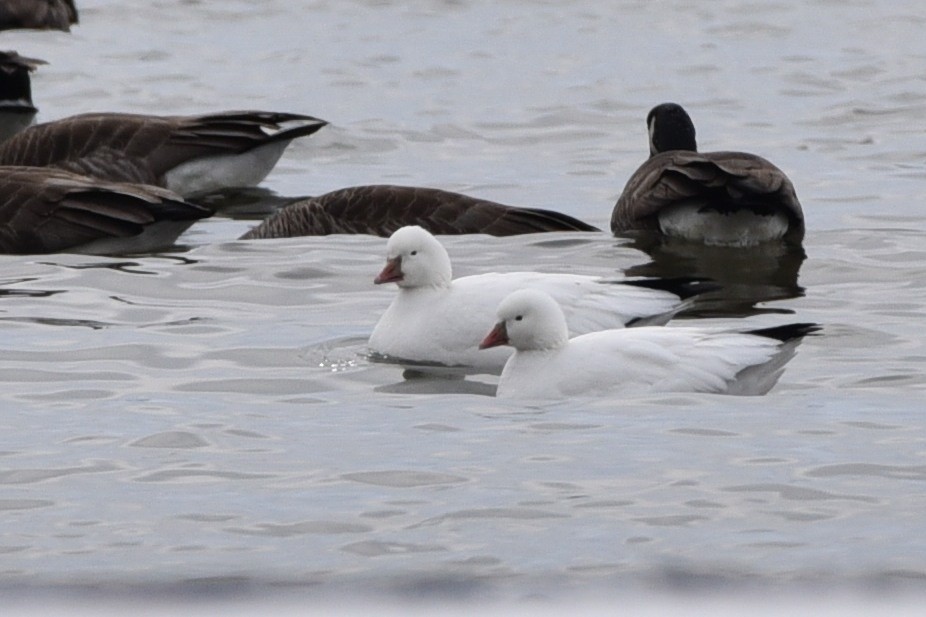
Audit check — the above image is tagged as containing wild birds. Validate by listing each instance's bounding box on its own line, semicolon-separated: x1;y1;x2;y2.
0;1;328;254
237;184;604;240
610;101;807;264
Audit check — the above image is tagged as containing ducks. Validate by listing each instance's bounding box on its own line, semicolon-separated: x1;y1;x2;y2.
367;224;683;368
480;287;823;401
